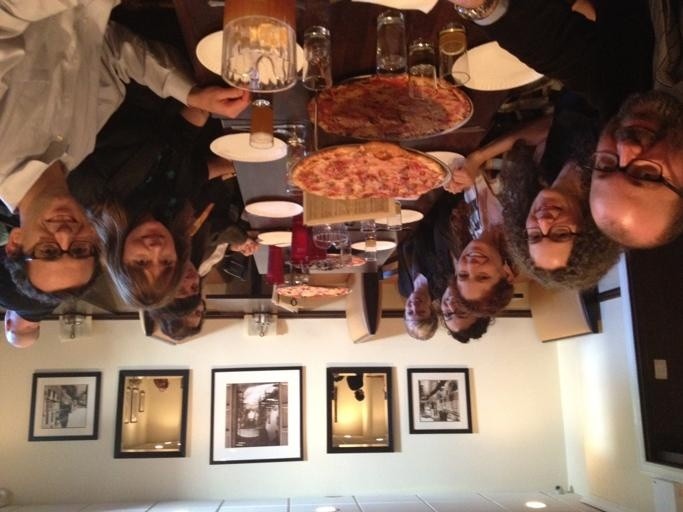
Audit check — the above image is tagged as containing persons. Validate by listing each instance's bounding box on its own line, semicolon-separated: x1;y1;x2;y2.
157;150;238;316
396;210;443;340
437;190;488;343
500;77;627;288
0;1;250;302
442;116;554;316
148;220;260;341
448;0;683;248
346;374;364;400
85;0;210;311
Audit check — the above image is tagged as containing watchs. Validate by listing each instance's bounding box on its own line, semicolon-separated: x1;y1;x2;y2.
454;1;497;21
